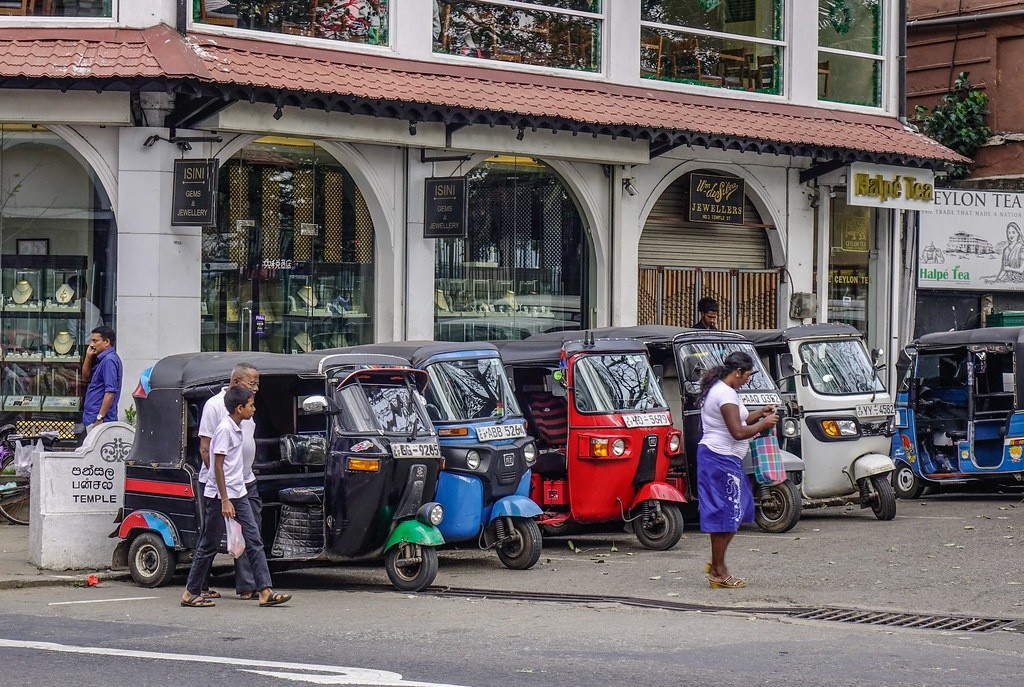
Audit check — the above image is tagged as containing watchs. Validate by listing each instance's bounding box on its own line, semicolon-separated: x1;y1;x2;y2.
97;414;104;421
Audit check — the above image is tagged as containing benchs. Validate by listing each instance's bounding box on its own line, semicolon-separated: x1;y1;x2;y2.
243;437;289;472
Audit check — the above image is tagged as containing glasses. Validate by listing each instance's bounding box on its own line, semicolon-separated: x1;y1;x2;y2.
236;377;260;386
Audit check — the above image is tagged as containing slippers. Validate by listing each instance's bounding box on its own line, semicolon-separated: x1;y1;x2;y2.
240;592;259;600
181;594;215;607
259;591;292;607
200;590;221;598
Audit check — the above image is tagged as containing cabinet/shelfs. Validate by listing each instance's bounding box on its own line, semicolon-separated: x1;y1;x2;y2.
0;254;88;446
282;260;373;353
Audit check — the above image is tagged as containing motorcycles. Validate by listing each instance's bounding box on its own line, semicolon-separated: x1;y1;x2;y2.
724;322;898;522
527;325;806;534
889;326;1024;501
108;348;446;592
314;340;544;572
481;339;690;550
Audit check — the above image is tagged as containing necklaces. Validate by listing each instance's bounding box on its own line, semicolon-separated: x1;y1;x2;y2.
57;338;70;344
58;287;67;301
16;286;31;296
299;335;310;346
301;292;314;301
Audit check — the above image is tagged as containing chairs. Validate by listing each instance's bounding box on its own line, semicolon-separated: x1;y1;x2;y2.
200;0;366;44
475;10;522;64
578;23;593;67
438;4;451;52
671;38;723;86
520;391;572;479
523;19;550;65
716;47;747;87
817;60;830;98
641;33;664;78
756;54;774;90
553;30;573;64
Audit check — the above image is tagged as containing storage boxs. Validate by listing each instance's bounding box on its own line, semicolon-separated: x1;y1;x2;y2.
542;481;569;506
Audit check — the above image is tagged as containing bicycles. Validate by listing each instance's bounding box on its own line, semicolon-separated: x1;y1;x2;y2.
0;424;61;525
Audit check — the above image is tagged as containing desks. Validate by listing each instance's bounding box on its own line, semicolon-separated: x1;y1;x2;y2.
569;43;578;46
501;21;549;62
700;50;746;86
642;43;660;50
740;69;830;79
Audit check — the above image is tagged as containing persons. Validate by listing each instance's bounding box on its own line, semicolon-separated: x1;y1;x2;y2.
67;275;104;355
204;0;247;29
197;362;263;600
181;386;293;607
691;297;718;329
696;352;780;589
82;326;123;435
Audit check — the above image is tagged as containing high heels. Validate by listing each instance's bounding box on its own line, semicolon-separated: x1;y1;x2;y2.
708;576;747;589
705;561;718;574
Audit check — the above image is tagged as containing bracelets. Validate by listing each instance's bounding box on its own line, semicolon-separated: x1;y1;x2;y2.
222;498;228;503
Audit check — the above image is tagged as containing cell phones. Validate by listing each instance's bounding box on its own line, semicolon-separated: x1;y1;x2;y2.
771;408;777;415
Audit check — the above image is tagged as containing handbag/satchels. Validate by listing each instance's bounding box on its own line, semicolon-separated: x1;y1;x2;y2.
749;417;788;487
14;438;45;478
224;515;246;559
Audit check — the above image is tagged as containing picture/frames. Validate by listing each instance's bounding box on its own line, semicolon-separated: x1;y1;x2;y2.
15;238;50;255
